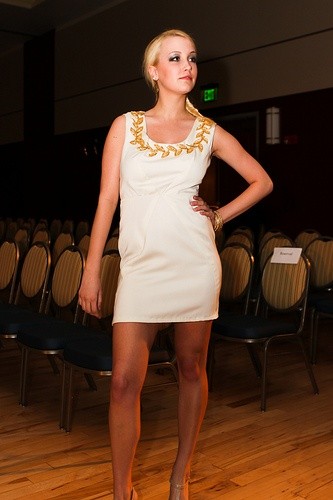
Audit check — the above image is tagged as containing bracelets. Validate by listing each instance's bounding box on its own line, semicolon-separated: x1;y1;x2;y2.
212;210;223;232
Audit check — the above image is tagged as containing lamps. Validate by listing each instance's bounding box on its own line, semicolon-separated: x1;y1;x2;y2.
265;106;280;145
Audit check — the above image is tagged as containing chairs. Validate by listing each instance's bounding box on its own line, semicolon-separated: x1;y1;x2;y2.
0;216;333;434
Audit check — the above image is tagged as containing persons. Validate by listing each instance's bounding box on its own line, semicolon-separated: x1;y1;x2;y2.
76;29;273;500
